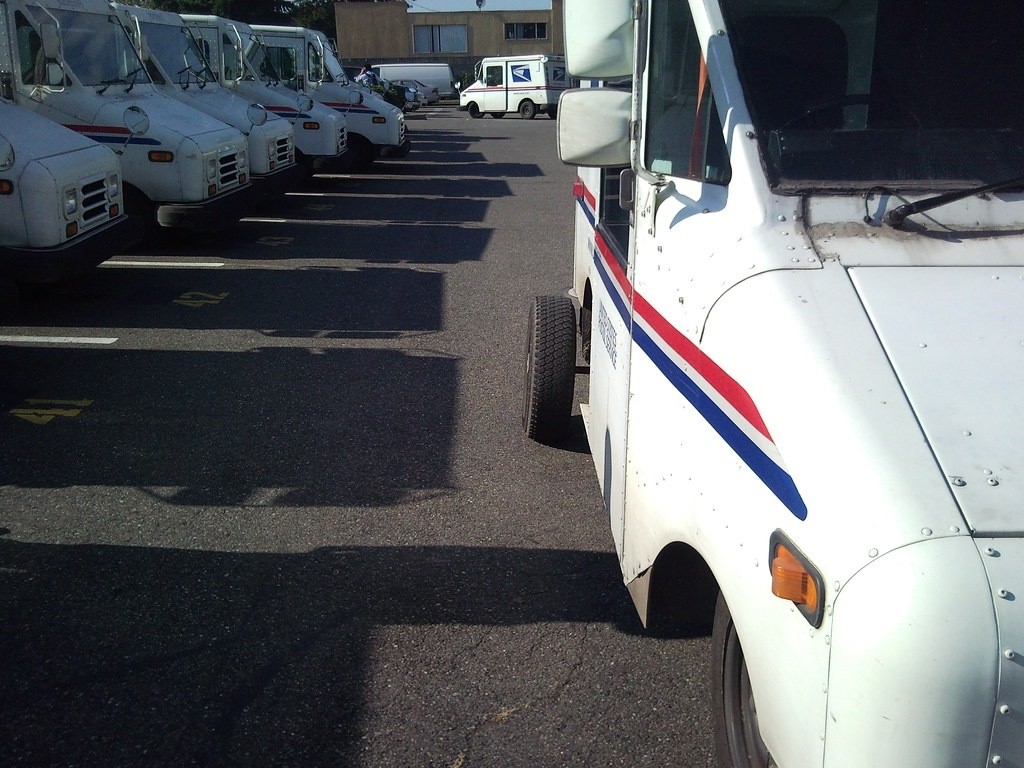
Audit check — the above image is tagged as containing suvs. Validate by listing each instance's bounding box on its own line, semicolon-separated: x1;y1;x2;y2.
343;66;421;113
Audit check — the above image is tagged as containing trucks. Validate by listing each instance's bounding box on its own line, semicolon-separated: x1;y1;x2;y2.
454;54;574;120
180;12;361;171
0;0;269;204
1;93;150;256
248;23;406;163
110;2;315;180
523;0;1024;768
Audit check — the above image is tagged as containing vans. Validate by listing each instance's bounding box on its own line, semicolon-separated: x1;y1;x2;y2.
369;63;455;98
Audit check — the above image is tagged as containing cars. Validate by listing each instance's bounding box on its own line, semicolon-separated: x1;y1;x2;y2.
390;77;440;103
